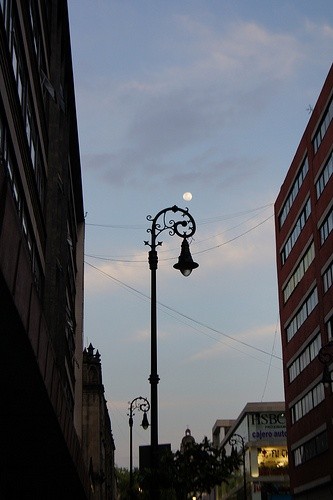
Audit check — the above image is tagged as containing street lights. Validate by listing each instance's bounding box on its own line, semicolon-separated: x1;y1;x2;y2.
228;433;247;500
143;203;199;500
126;396;150;499
